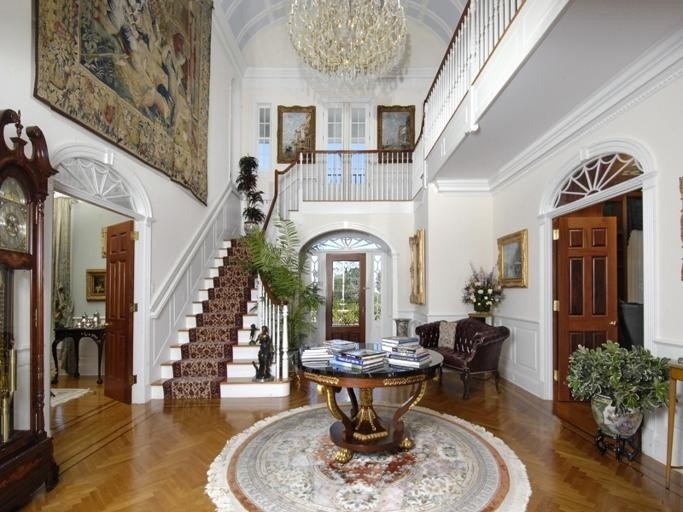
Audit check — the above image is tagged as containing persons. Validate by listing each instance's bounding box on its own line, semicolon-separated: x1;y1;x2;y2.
249;324;273;378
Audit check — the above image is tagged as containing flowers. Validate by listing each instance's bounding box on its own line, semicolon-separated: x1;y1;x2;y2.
463;262;505;310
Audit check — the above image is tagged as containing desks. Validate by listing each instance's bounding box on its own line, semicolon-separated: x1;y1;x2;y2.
52;328;106;384
303;349;444;463
467;313;495;326
665;364;683;490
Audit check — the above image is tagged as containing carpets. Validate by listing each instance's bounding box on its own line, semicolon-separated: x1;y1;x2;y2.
203;402;533;512
51;388;92;407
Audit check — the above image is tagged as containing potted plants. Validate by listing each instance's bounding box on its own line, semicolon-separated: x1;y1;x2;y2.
565;341;677;439
237;155;266;235
241;217;325;373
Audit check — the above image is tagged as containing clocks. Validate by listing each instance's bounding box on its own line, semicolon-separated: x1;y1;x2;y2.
0;108;60;512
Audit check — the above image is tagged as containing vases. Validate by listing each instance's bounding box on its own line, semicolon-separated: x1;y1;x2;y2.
474;303;490;312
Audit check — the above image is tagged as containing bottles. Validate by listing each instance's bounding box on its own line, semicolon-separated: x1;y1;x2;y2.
73;311;100;327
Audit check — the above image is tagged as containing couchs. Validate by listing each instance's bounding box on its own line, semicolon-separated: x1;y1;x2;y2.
414;319;510;401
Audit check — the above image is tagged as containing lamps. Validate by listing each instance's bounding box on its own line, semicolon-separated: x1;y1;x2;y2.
285;0;408;85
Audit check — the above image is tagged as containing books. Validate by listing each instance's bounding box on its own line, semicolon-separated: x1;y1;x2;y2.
300;336;433;374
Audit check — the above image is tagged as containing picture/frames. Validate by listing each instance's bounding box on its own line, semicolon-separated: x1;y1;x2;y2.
377;104;415;163
86;268;106;301
409;229;425;306
277;104;316;164
497;229;528;288
